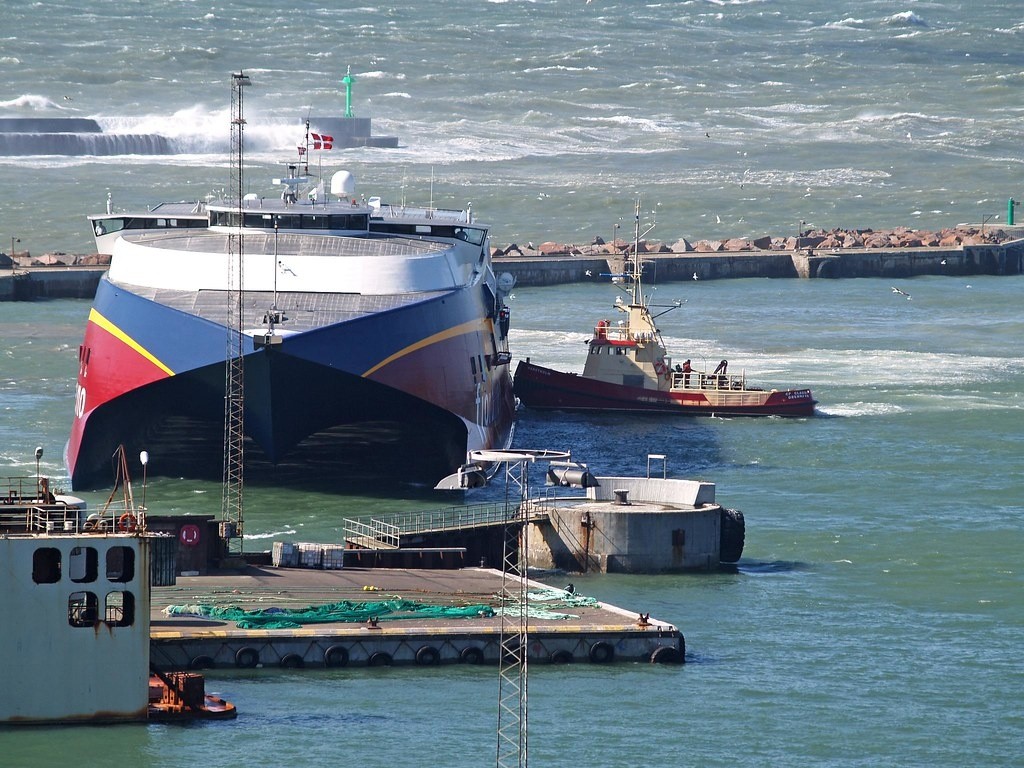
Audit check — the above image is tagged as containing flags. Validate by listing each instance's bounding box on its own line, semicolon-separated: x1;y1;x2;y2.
298;147;304;155
311;133;334;149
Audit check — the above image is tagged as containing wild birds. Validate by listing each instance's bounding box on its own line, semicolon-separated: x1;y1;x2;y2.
515;122;929;299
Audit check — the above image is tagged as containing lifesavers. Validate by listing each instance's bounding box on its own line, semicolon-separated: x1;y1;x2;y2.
282;652;305;668
192;654;214;668
325;645;349;667
236;646;259;667
87;513;102;530
98;520;108;531
372;650;393;665
651;646;681;661
654;361;667;376
553;649;573;661
590;641;613;664
417;645;440;667
119;514;136;532
502;642;522;665
461;646;485;665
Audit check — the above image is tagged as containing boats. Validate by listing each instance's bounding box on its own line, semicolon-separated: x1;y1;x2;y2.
296;66;400;151
58;70;520;507
508;197;821;419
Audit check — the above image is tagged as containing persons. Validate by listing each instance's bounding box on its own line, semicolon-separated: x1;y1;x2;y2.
95;221;106;236
310;198;315;205
683;359;691;388
